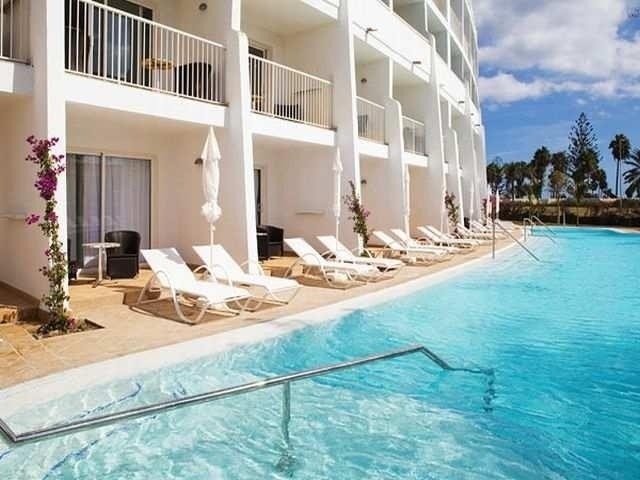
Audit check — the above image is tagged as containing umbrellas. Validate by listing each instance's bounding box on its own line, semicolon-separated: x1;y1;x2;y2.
440;168;447;234
495;188;500;220
402;163;412;235
468;176;474;231
198;126;224;243
330;146;344;241
485;183;491;226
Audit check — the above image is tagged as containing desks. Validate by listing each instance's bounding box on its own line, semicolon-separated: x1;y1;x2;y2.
81;242;121;288
141;57;173;88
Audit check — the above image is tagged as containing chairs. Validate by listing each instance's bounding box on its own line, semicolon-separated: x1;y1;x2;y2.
173;61;369;136
105;230;141;280
281;237;376;290
136;246;253;326
190;242;304;308
456;218;513;242
371;228;459;267
416;226;485;254
316;234;406;281
